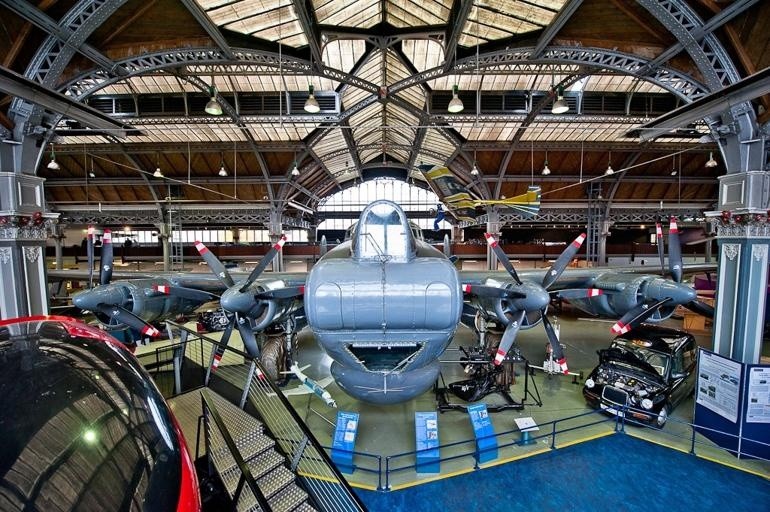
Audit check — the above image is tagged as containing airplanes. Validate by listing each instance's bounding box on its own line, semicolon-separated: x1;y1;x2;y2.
48;198;715;404
418;162;542;221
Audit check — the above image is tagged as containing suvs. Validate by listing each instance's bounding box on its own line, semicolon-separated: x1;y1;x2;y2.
581;324;699;429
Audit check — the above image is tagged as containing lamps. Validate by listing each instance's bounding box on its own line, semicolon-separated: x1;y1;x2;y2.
605;151;613;176
304;50;320;112
153;152;163;177
552;85;569;114
89;158;94;178
219;151;227;177
205;75;223;115
671;153;676;176
706;150;717;167
291;152;299;176
542;151;550;175
448;37;464;113
470;152;478;175
47;145;59;170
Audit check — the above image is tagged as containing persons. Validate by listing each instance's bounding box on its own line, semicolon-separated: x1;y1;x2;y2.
123;237;140;249
81;237;103;249
